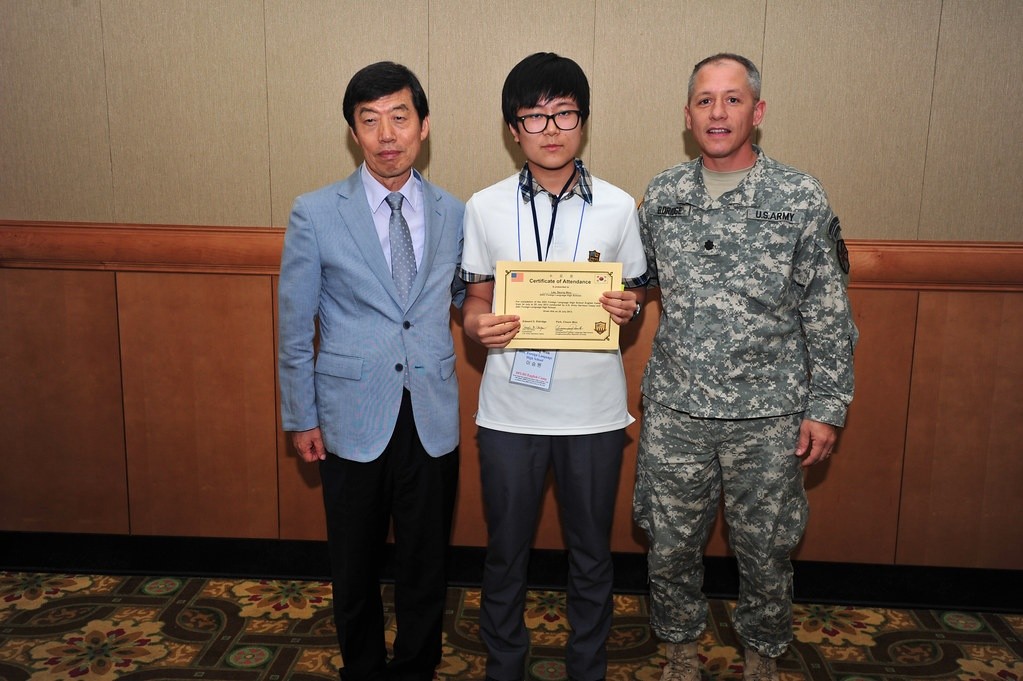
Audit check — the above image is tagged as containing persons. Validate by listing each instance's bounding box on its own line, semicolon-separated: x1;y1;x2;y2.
275;61;467;681
459;50;651;681
632;54;858;681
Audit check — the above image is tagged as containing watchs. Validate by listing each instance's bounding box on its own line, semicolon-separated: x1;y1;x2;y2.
630;301;642;321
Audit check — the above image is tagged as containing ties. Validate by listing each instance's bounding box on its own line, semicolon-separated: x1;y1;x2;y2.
385;192;418;390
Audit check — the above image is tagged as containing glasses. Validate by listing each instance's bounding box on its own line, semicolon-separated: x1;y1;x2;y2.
516;110;583;134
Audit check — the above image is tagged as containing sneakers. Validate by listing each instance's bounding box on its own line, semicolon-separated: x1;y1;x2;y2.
660;642;702;681
744;647;780;681
